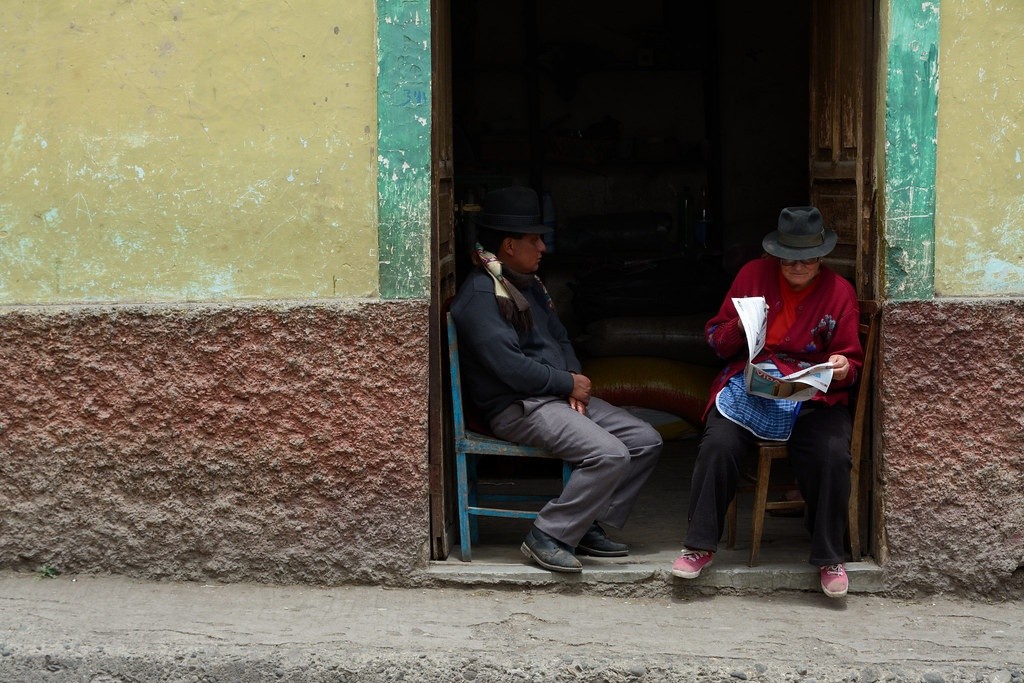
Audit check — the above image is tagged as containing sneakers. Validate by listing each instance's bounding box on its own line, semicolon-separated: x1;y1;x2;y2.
671;546;714;579
818;563;850;599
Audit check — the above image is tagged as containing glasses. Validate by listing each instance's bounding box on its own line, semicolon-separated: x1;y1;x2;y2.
780;258;821;266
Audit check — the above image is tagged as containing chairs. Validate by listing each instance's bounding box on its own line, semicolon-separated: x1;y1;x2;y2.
726;297;882;568
446;309;573;562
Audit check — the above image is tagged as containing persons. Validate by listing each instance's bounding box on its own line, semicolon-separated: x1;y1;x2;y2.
666;205;865;598
448;191;663;572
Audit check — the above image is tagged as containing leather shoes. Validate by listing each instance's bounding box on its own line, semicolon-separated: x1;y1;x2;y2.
520;525;583;573
579;520;630;556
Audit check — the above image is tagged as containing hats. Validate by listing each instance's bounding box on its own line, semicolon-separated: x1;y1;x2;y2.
762;206;839;261
477;185;553;234
